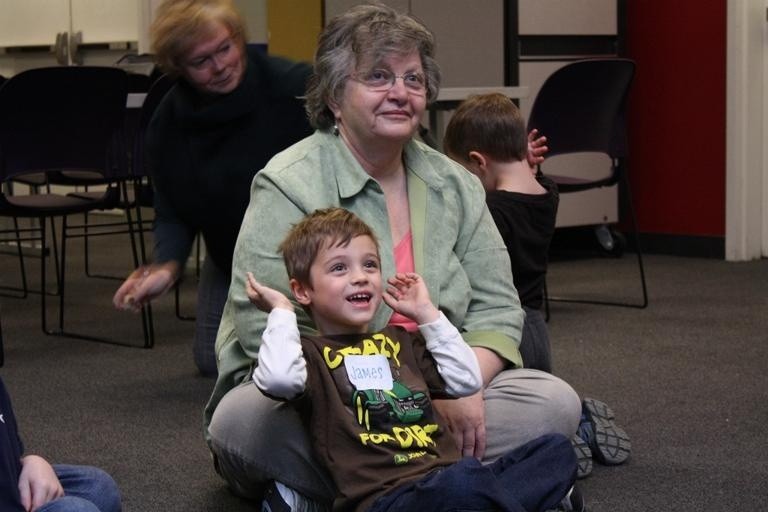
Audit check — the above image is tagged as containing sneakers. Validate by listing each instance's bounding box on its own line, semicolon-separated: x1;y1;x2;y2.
576;398;634;465
569;433;597;480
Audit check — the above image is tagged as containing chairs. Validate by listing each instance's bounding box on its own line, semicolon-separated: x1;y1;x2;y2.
0;58;639;350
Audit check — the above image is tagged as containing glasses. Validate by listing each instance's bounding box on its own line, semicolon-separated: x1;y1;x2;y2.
344;67;431;92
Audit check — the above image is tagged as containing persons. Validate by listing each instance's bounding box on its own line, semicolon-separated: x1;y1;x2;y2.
441;90;632;482
0;333;123;511
244;203;581;510
110;1;437;382
197;4;590;511
